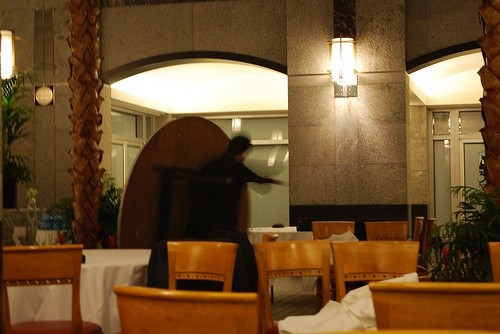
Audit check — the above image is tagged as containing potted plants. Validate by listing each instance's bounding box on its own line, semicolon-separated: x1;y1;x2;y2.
417;154;500;283
0;61;47;211
36;199;74;245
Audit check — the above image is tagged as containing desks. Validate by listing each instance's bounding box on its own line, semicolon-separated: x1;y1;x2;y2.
248;224;323;319
6;249;152;334
117;117;286;250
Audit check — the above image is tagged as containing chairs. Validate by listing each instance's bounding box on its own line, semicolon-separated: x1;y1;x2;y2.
252;239;331;331
369;280;500;333
166;241;239;292
365;221;409;241
0;244;104;334
311;222;356;241
111;287;259;334
330;240;421;303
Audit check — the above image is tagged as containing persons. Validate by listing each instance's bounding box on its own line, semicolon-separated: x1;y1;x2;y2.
186;135;279;290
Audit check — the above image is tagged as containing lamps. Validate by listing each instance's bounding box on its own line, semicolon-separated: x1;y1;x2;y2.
329;35;359;97
